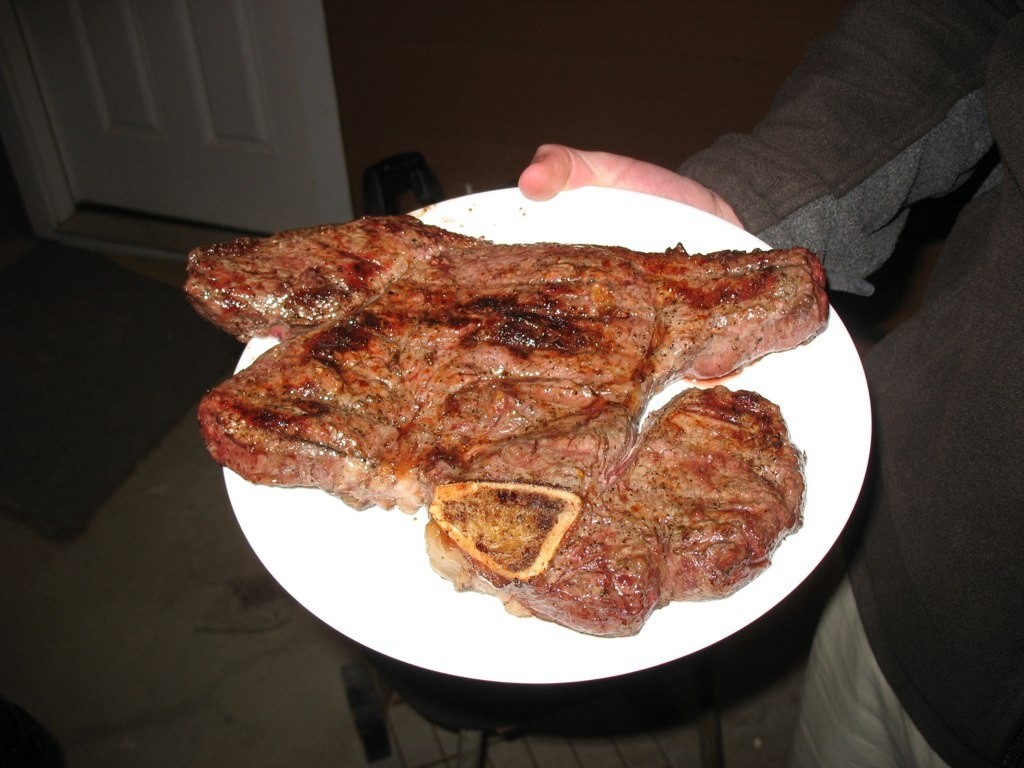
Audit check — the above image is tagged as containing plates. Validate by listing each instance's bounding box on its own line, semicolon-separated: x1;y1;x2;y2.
223;184;873;685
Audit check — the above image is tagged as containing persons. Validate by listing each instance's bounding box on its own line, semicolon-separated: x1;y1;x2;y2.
517;1;1024;768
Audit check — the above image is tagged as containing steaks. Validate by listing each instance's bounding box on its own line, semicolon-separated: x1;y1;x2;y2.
182;214;828;639
